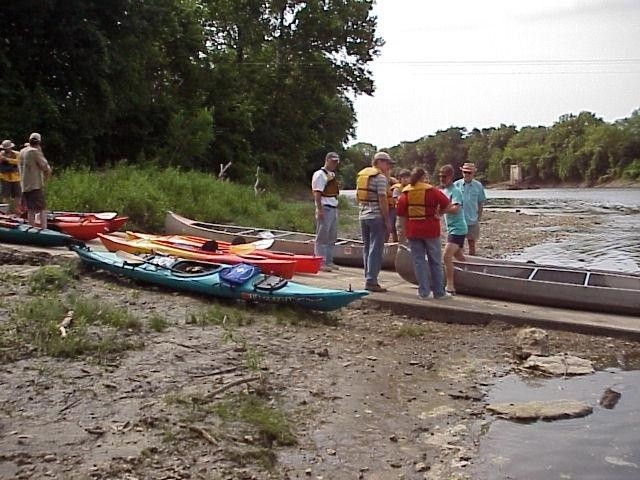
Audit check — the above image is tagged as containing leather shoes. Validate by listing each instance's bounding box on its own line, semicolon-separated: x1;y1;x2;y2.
365;283;387;292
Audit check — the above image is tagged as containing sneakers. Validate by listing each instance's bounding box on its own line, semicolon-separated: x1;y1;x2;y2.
327;264;339;270
419;294;433;299
435;292;452;299
320;265;331;272
446;289;456;296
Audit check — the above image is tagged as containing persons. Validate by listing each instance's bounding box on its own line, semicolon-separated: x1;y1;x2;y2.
355;151;488;300
0;139;21;217
16;132;53;230
312;151;343;272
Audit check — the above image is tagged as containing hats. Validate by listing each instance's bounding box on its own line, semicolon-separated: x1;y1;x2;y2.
326;152;339;161
439;164;454;177
29;133;42;142
374;152;396;164
460;163;478;173
1;140;16;149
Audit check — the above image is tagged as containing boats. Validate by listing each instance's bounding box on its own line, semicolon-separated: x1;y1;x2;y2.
164;209;398;270
0;205;133;241
67;244;375;312
0;223;92;250
124;230;325;277
392;239;640;317
97;232;298;283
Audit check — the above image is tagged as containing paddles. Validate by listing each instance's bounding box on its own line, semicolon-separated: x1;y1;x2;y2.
219;239;275;256
117;250;184;274
54;212;117;220
